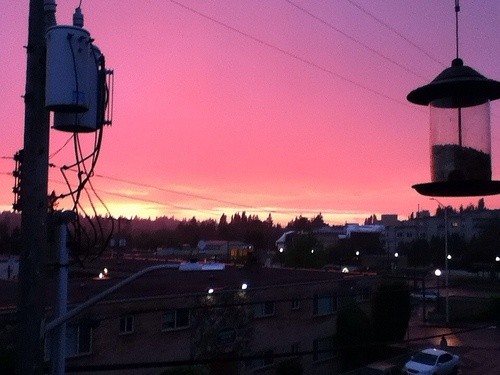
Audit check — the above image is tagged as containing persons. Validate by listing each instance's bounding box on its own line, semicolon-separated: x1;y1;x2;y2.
440;336;447;347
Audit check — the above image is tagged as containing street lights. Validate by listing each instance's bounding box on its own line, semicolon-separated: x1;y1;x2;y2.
280;248;500;277
15;262;225;375
434;269;442;312
430;197;449;324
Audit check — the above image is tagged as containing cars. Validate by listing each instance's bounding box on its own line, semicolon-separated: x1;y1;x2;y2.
405;349;460;375
